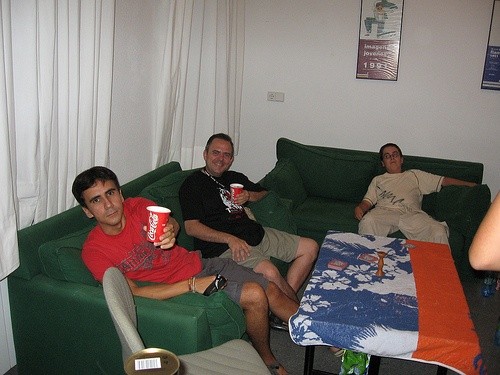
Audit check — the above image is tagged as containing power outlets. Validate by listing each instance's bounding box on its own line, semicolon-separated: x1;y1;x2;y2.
266;92;284;101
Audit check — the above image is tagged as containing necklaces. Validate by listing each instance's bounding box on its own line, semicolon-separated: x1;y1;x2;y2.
203;166;225;188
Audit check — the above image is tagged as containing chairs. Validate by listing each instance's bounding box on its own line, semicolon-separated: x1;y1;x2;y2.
103;265;273;375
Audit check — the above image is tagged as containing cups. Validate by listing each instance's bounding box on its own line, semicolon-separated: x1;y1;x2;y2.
230;183;244;204
146;206;171;242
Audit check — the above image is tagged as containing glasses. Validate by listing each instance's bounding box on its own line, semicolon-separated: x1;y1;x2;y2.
381;153;400;159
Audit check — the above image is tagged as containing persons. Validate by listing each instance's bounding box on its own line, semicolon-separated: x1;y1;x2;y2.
71;165;346;375
355;143;479;253
469;189;500;271
179;133;318;331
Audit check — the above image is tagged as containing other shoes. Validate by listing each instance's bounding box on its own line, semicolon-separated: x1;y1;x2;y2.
336;348;345;357
268;363;288;375
268;312;289;331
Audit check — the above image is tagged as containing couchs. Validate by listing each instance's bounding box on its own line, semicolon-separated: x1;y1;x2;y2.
249;136;492;284
8;161;292;375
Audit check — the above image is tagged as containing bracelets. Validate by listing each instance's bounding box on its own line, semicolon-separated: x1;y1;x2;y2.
188;277;196;293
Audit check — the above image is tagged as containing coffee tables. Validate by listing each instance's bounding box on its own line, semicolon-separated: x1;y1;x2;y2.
289;229;486;375
